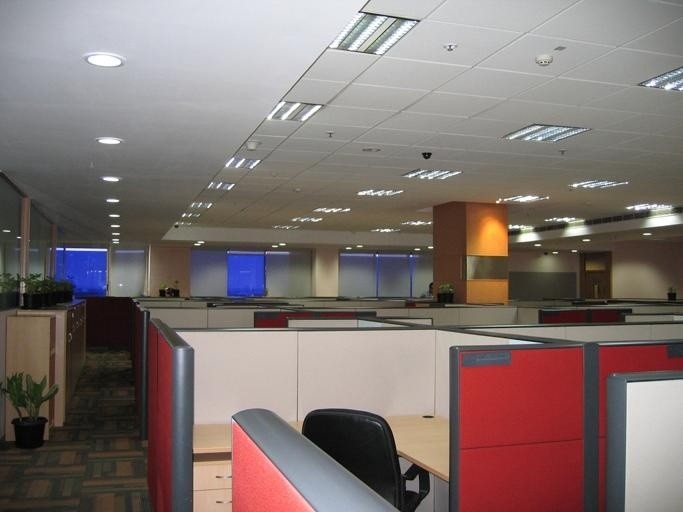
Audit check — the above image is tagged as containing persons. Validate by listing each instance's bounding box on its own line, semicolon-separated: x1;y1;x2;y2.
425;282;433;297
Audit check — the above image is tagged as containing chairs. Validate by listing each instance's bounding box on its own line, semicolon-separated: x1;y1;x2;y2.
300;407;430;511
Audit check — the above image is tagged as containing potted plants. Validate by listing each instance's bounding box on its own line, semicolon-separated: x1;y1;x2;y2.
14;270;75;309
0;371;59;449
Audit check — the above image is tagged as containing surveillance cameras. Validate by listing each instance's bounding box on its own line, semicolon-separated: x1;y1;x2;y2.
421;152;432;160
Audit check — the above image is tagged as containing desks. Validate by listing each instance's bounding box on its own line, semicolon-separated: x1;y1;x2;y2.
192;411;450;512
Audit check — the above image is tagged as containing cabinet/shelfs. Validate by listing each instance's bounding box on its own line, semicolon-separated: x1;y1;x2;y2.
15;299;86;426
